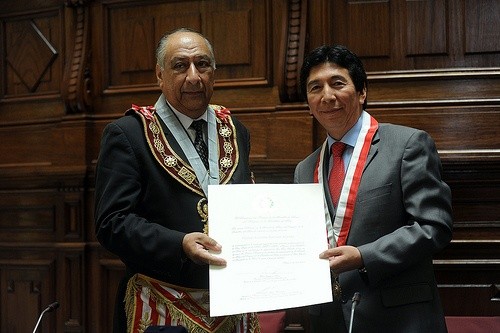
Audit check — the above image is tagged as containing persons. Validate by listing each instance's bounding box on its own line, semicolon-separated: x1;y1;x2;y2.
93;27;260;333
294;43;455;333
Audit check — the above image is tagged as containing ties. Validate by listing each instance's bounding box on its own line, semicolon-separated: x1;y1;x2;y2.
190;119;209;172
328;141;345;211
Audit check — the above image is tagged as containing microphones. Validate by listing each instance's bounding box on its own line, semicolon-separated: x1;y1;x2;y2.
348;292;361;333
33;302;60;333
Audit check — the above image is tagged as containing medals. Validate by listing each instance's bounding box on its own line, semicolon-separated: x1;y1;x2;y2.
332;280;343;301
